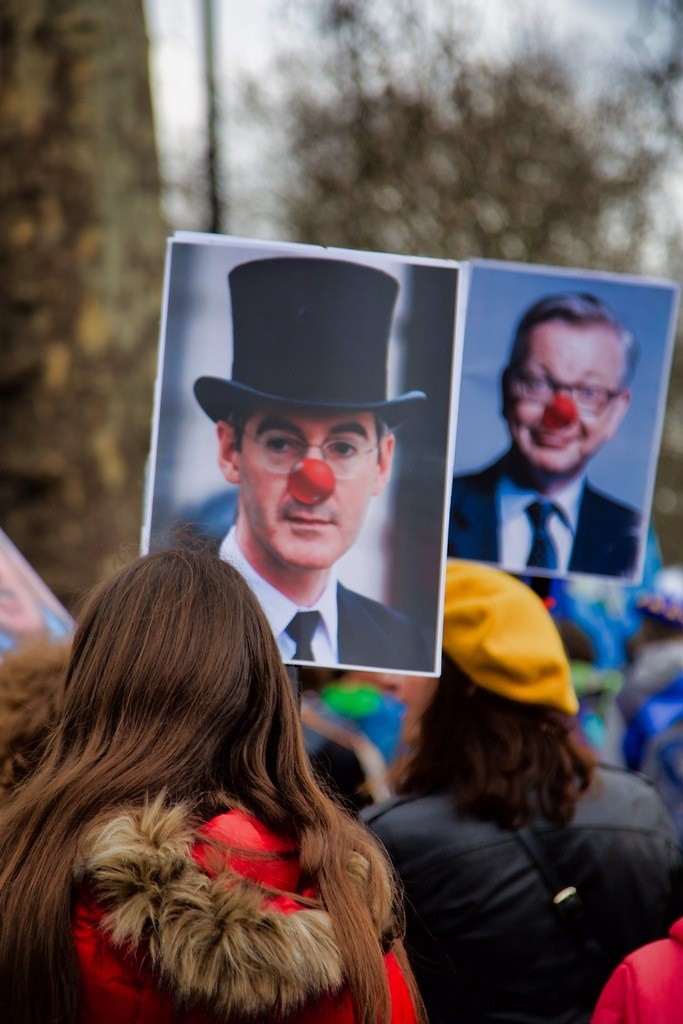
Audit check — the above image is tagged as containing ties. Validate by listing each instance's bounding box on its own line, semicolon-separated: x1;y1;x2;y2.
284;610;322;660
523;500;558;571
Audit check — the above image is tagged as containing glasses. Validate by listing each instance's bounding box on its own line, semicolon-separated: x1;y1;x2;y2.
512;373;622;411
240;429;382;479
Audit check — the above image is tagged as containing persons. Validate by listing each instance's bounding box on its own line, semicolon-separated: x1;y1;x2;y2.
0;550;683;1024
182;256;432;670
446;289;644;579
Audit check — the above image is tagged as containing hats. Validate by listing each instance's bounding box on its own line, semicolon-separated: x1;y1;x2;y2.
439;555;578;713
194;255;428;432
